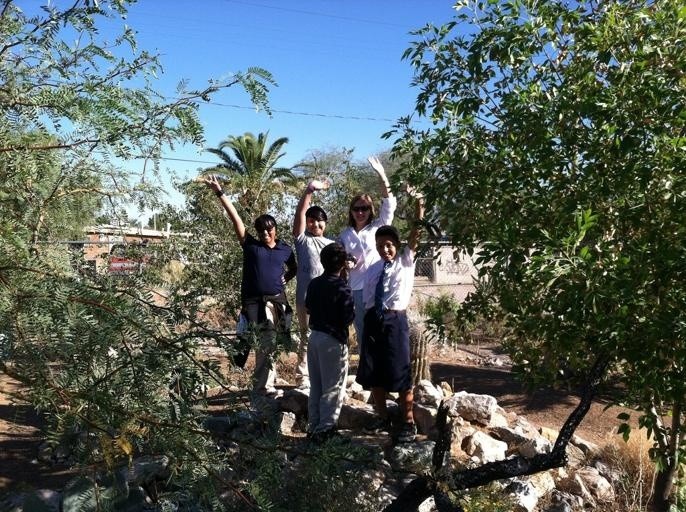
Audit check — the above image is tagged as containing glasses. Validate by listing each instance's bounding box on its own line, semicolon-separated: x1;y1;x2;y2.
351;205;370;212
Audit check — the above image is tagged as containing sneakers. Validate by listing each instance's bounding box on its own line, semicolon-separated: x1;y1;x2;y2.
398;422;418;443
307;430;350;449
362;417;391;434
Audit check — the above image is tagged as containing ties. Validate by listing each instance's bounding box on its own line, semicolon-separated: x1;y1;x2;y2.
375;261;391;322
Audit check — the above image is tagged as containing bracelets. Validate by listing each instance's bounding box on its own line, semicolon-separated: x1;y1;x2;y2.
307;184;314;192
215;190;225;197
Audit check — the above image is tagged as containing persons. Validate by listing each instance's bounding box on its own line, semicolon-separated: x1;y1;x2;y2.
339;153;398;356
303;242;357;439
204;174;296;373
354;184;428;442
293;178;342;391
